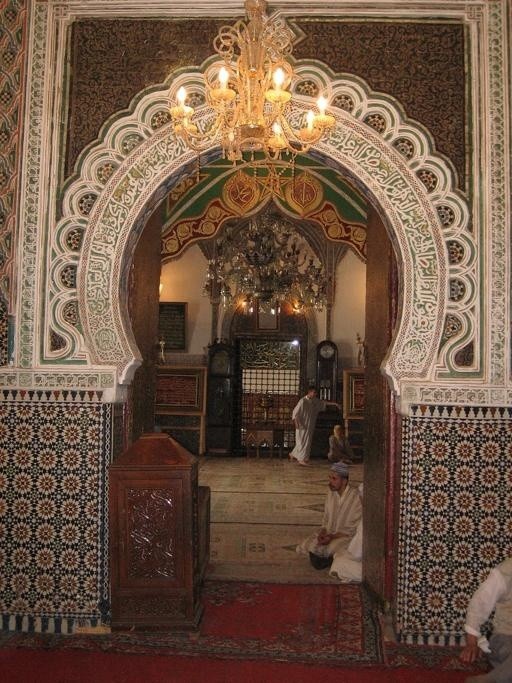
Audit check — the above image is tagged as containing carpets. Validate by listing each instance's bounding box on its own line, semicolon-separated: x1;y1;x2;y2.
0;577;387;667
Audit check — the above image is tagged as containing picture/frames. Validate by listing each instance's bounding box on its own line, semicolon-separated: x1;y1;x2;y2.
153;365;207;415
342;368;364;419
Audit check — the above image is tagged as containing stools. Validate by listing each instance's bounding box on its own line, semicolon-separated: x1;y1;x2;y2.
243;428;286;464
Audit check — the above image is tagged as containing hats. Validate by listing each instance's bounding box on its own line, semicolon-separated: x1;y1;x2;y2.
330;460;350;477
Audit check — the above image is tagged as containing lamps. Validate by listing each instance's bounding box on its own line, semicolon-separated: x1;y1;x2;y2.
198;205;332;316
166;0;330;203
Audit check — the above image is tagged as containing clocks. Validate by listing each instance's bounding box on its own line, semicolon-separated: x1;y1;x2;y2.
315;339;339;402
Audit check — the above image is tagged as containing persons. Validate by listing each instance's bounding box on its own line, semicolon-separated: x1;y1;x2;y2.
461;555;511;683
328;425;354;463
329;482;364;584
289;386;342;467
309;462;363;570
